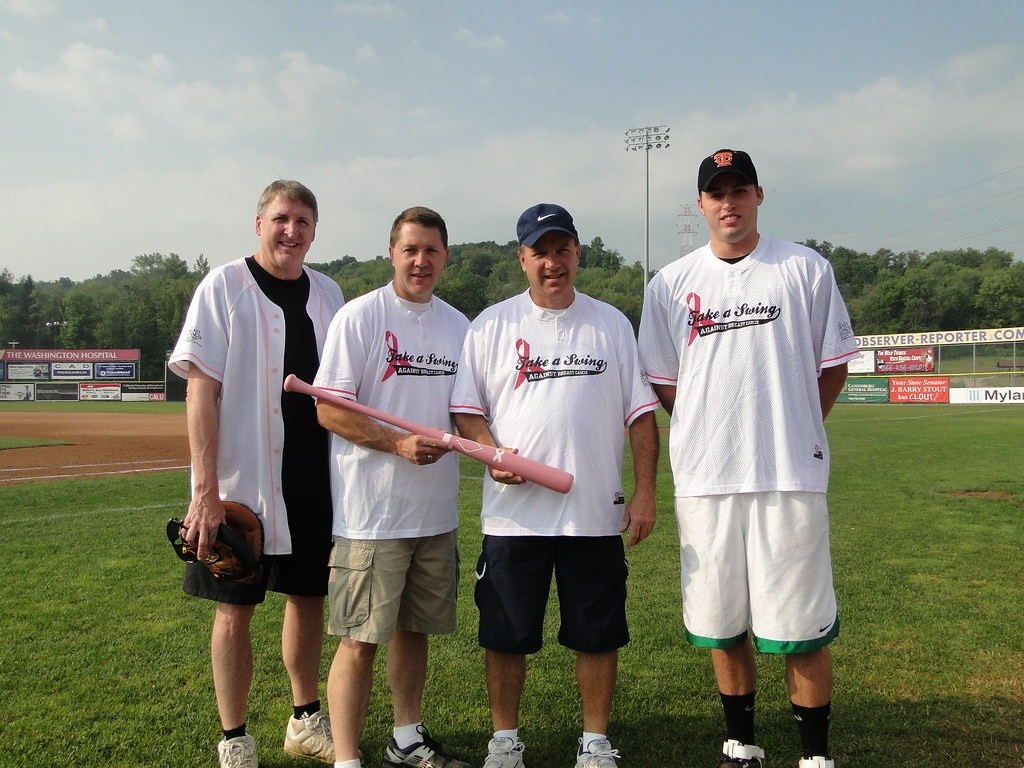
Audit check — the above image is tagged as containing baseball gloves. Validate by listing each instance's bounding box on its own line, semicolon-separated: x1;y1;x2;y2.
163;500;267;587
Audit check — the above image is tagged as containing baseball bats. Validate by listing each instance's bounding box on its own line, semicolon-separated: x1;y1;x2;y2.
283;374;575;495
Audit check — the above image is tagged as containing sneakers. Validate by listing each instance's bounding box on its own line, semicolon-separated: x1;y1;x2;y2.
283;709;364;765
382;724;471;768
217;731;259;768
718;738;766;768
483;736;525;768
574;737;621;768
798;755;834;768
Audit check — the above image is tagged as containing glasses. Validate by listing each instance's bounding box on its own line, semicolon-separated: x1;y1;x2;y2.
166;518;200;563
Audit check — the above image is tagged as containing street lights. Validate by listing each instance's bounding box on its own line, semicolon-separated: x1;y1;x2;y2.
623;125;673;296
46;320;67;349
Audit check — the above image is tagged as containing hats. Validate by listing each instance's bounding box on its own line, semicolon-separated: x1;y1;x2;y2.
697;148;758;195
516;203;580;246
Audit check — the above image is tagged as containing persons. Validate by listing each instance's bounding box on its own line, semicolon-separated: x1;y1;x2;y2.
448;204;659;768
637;149;862;768
312;207;471;768
169;180;346;768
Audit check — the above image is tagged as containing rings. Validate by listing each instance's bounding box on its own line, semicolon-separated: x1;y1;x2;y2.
181;525;188;531
427;455;433;462
